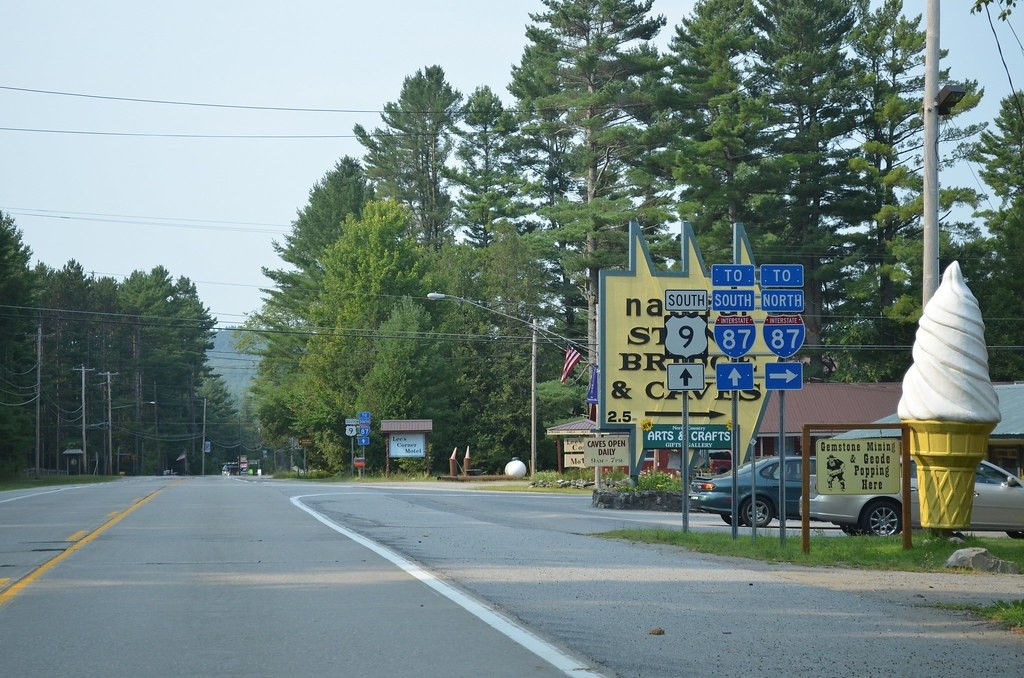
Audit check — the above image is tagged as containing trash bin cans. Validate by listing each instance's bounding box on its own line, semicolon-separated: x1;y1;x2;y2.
63;449;84;476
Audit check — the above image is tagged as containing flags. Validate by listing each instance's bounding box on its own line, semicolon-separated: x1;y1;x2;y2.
561;344;582;383
586;365;598;404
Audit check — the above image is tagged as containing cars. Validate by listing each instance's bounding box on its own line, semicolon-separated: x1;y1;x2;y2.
689;455;802;527
799;455;1024;540
222;465;237;476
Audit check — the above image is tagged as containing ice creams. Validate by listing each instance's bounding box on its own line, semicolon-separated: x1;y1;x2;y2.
897;261;1001;528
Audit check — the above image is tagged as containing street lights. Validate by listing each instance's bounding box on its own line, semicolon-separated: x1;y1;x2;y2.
108;401;156;476
922;84;967;310
427;292;603;491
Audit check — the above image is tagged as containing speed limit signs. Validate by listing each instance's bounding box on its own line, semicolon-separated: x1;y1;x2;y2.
763;315;805;359
714;315;757;358
360;425;371;435
